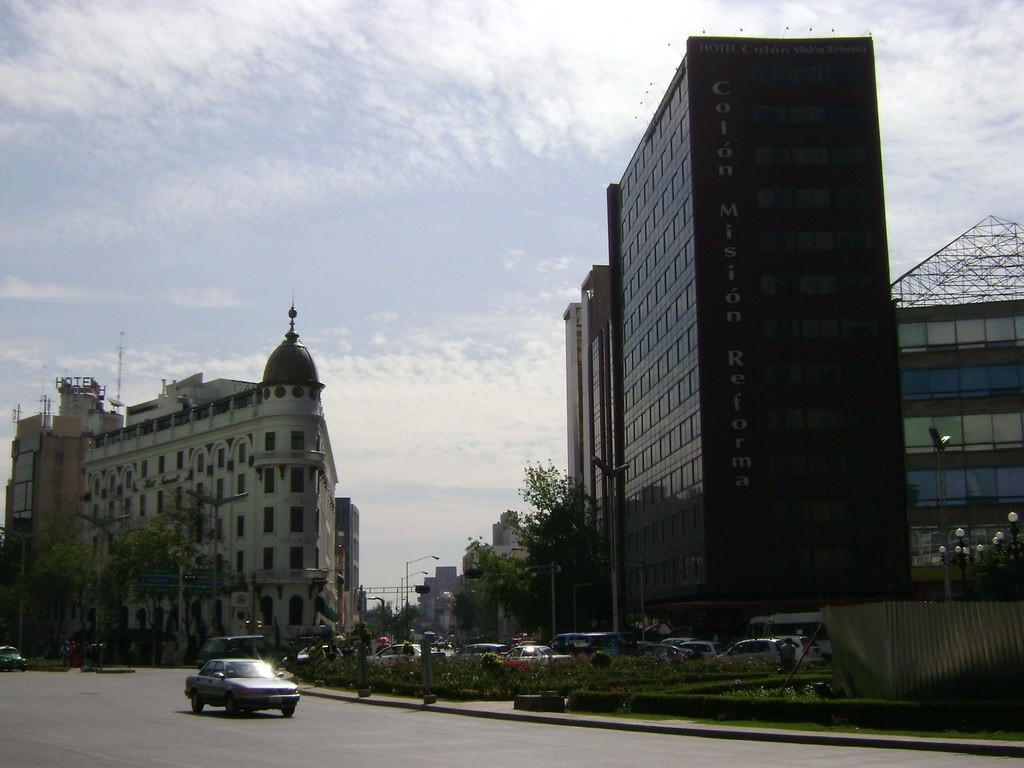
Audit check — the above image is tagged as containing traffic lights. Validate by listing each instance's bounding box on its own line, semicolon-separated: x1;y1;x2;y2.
255;621;262;630
244;620;252;629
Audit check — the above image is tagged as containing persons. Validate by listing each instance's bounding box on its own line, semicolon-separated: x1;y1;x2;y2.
376;639;389;657
444;641;449;651
779;638;795;674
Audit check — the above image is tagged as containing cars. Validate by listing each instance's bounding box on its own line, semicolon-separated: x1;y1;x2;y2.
517;641;539;648
498;645;572;668
661;638;725;661
366;643;451;668
0;645;28;672
185;657;301;718
632;643;697;663
774;634;826;668
444;643;509;667
713;637;827;677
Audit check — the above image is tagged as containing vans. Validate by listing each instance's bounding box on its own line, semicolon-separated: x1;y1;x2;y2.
543;633;638;659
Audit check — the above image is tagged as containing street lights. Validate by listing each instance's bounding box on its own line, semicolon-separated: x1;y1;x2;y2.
573;582;594;632
401;571;428;612
369;596;386;635
406;555;440;641
